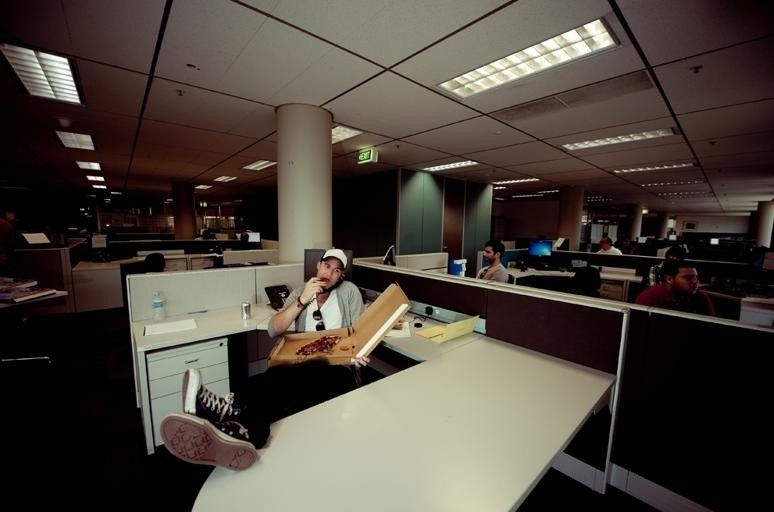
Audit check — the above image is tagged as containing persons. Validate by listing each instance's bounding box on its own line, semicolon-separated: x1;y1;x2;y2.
0;210;30;278
475;240;509;282
232;232;254;251
597;234;639;254
160;247;373;470
46;222;80;248
635;259;717;317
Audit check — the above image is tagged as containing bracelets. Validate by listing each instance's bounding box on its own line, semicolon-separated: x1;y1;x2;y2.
297;296;306;308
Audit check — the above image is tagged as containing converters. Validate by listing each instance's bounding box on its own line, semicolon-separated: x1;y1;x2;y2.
425;307;433;315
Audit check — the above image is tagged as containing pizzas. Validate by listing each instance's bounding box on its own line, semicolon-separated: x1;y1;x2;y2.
296;335;342;355
314;278;329;283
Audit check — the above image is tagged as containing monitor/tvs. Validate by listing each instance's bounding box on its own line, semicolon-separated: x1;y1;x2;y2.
669;235;677;240
15;230;49;248
383;245;397;266
91;233;107;249
709;238;720;246
200;229;208;235
557;238;569;250
637;237;647;244
761;248;774;271
527;240;552;257
240;232;261;250
304;248;354;282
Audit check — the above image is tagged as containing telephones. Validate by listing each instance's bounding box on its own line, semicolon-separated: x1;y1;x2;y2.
323;271;351;292
517;259;527;271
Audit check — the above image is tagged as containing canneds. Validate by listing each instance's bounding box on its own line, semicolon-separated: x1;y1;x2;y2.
241;302;250;320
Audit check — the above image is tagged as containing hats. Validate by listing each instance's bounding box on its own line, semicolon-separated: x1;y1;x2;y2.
323;247;347;269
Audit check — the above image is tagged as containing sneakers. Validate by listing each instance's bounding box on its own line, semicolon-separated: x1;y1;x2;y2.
160;369;258;471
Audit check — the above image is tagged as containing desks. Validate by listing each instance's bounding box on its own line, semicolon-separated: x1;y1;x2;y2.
695;278;773;327
0;289;68;368
130;302;616;511
504;265;643;303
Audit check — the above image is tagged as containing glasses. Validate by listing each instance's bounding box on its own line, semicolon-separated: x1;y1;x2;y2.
313;308;325;332
480;268;487;279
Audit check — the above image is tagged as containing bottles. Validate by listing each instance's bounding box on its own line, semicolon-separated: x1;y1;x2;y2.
153;291;164;320
